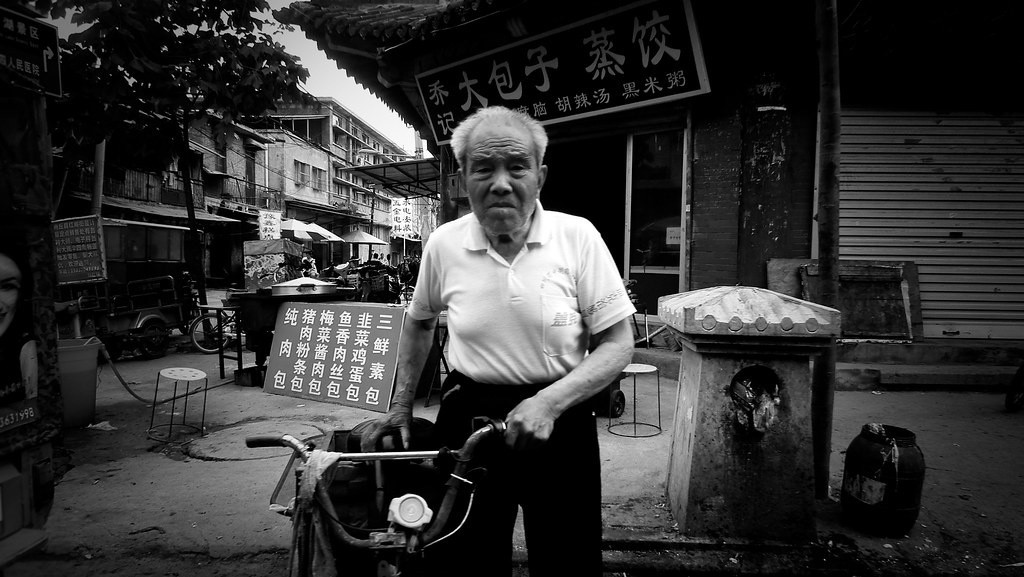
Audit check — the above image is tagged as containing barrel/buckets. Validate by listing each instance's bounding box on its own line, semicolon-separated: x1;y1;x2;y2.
840;425;924;539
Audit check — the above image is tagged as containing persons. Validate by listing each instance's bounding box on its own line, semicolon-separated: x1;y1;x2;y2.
305;252;316;277
0;251;37;403
361;107;637;577
372;253;387;264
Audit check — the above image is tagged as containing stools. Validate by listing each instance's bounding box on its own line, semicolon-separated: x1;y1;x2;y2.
147;367;208;444
608;364;662;438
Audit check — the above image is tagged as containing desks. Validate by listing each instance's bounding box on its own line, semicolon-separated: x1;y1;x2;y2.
197;305;246;380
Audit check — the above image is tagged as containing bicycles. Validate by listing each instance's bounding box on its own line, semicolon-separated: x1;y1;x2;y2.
188;303;245;354
244;414;508;577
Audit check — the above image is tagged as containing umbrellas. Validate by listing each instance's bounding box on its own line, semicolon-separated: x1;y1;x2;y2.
343;228;388;257
279;218;345;244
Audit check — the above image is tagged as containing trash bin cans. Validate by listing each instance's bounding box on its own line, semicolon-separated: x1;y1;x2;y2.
657;284;842;542
